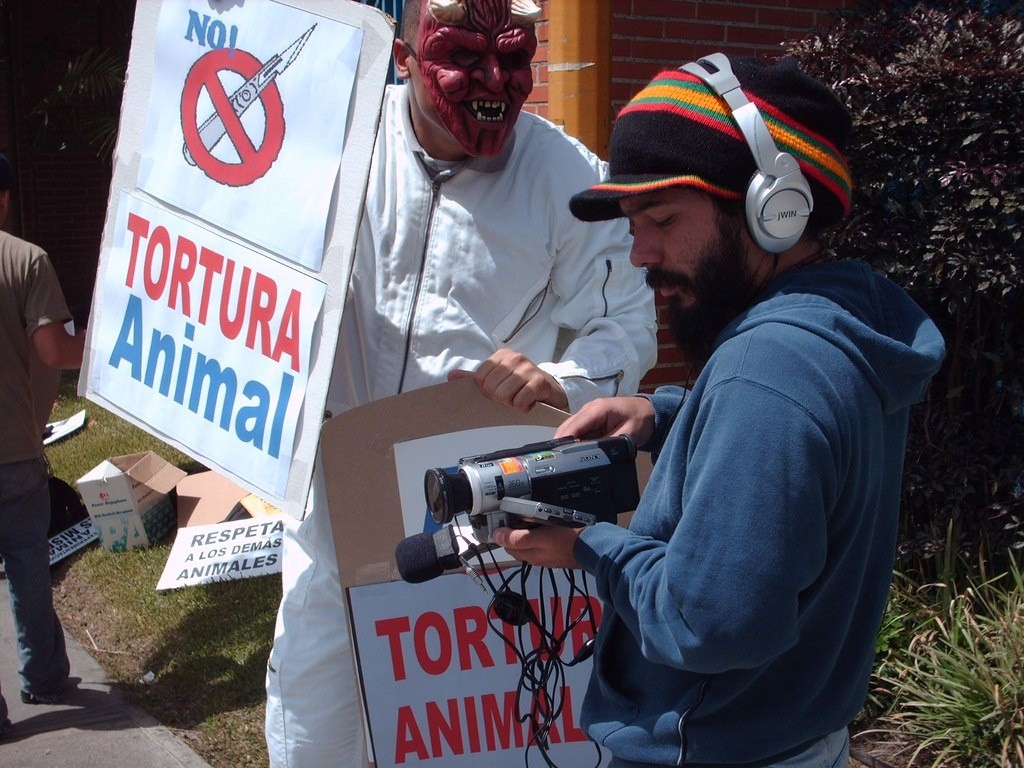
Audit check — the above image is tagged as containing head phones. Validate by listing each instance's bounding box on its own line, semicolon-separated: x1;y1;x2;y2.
676;52;814;255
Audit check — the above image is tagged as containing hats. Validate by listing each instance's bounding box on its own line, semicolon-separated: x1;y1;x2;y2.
567;56;855;223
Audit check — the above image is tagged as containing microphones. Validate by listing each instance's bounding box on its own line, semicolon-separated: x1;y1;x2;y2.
395;523;504;583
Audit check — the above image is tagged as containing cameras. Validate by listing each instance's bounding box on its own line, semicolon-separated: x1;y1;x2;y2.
423;431;642;544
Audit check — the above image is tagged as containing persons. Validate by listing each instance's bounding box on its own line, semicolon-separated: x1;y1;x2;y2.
0;153;87;738
492;53;948;768
265;0;659;768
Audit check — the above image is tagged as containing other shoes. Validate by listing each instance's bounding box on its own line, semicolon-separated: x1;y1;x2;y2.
20;691;39;704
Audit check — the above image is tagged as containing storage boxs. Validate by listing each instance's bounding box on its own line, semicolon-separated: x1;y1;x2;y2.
74;450;188;556
